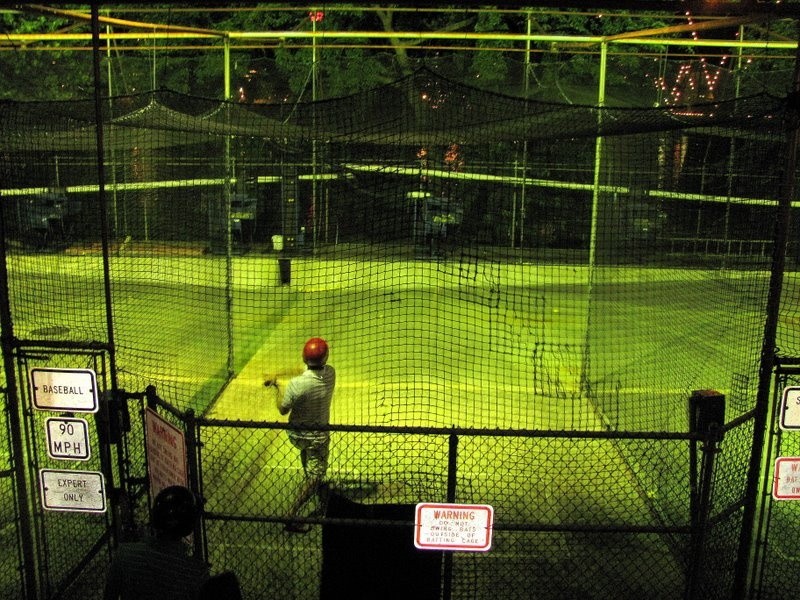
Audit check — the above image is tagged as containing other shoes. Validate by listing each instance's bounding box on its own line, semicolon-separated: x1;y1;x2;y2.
285;516;312;532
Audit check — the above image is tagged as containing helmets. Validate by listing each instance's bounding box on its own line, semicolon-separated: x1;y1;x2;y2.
302;337;329;367
151;485;207;522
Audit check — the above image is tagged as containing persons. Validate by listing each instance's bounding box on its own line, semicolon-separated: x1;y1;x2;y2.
101;485;242;600
263;338;336;536
